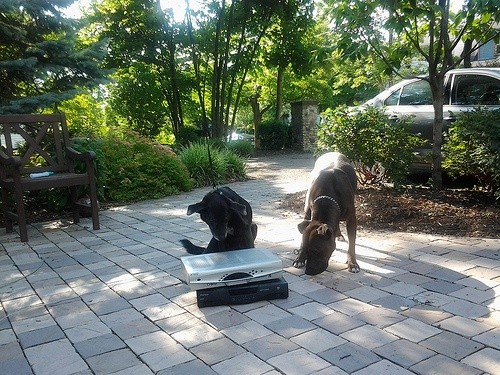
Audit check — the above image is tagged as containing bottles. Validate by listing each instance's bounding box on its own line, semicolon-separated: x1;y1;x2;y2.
30;172;54;178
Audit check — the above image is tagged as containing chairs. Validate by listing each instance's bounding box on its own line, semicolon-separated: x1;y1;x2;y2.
0;111;101;242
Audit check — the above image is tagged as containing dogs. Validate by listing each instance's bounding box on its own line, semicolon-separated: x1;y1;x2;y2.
179;187;258;255
292;151;360;276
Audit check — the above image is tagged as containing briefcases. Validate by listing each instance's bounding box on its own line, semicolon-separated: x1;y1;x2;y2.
180;247;281;291
197;277;288;308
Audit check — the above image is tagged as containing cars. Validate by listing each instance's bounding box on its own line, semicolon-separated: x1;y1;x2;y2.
222;132;255;143
320;68;500;184
